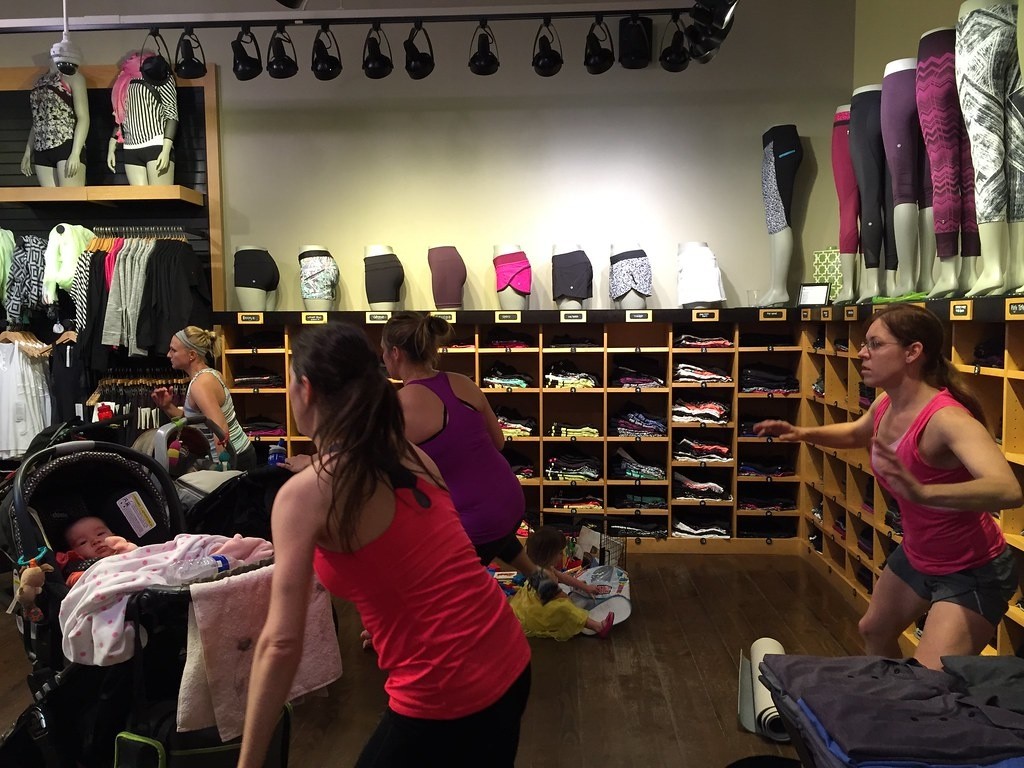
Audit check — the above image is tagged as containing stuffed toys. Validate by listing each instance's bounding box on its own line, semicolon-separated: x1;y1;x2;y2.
16;563;54;606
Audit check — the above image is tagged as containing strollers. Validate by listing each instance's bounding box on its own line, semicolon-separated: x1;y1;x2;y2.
129;415;296;545
0;439;340;768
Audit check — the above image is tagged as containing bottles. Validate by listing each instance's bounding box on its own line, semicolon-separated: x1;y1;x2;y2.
267;438;286;469
165;552;246;587
97;403;114;421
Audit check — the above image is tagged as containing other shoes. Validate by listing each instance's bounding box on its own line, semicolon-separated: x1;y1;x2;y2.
526;563;562;603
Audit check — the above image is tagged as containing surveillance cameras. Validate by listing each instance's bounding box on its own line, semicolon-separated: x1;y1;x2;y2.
50;42;82;75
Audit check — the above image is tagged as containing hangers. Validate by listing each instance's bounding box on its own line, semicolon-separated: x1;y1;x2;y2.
84;227;192;252
0;317;54;358
85;363;190;407
39;320;78;356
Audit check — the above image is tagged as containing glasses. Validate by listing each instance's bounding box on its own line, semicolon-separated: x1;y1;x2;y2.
858;337;906;352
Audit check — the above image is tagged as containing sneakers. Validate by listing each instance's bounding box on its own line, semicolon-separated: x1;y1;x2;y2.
595;611;614;638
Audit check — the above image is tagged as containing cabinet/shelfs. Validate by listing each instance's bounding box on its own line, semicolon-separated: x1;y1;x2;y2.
221;310;803;557
798;301;1023;673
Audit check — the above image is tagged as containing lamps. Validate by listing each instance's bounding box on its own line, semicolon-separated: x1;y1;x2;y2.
138;28;172;87
402;20;436;80
581;16;616;75
616;14;653;69
532;18;563;78
362;22;394;80
174;27;207;79
266;24;298;80
468;20;501;76
659;0;739;73
310;24;343;82
230;24;263;82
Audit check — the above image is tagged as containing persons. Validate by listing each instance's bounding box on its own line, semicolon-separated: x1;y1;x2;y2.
381;313;561;607
492;244;531;310
753;303;1024;672
510;527;615;643
955;0;1024;296
107;51;179;185
552;243;593;310
916;26;981;298
609;238;652;309
849;83;898;304
881;58;937;298
234;246;280;311
363;246;404;311
757;124;804;306
298;245;339;311
676;241;727;309
65;515;139;586
151;326;256;471
831;105;866;303
237;320;533;768
428;246;467;310
20;58;90;187
